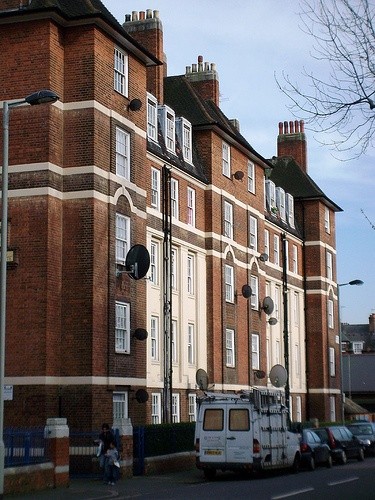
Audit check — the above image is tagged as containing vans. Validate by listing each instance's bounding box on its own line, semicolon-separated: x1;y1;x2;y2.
193;387;302;479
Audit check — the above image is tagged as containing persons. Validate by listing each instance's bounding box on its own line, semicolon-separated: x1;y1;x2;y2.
93;424;118;485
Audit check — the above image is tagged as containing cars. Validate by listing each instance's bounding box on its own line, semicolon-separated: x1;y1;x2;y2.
313;425;366;465
299;428;332;471
346;421;375;455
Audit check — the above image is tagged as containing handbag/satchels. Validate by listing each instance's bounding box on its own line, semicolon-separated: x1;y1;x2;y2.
113;460;120;468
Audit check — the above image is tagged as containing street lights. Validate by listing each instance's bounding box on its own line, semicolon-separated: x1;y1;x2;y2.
0;91;62;496
336;279;366;427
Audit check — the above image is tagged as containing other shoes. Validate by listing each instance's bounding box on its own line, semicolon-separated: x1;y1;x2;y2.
108;481;114;485
